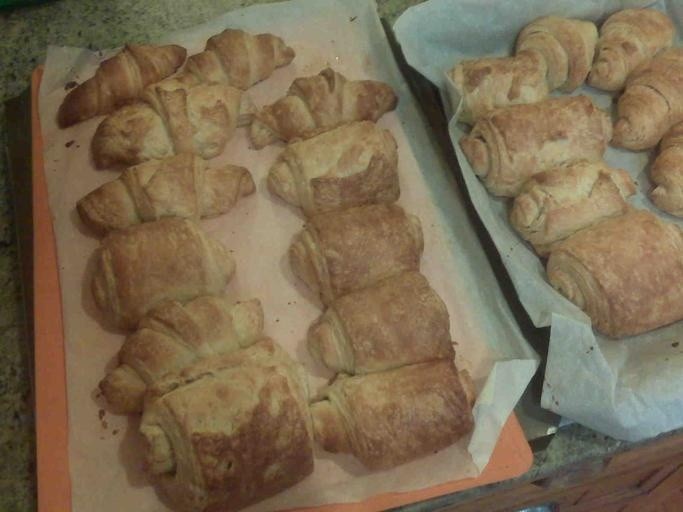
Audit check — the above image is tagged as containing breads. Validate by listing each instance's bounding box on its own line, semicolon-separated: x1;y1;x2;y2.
547;209;682;342
307;271;456;376
444;49;551;125
311;360;477;468
90;210;233;332
90;79;258;171
160;28;296;93
265;119;400;217
585;8;674;90
74;153;258;230
138;362;316;512
288;202;426;307
645;123;682;218
508;159;638;258
516;12;599;96
58;43;188;127
249;69;399;148
100;297;265;413
612;45;681;150
460;96;611;200
